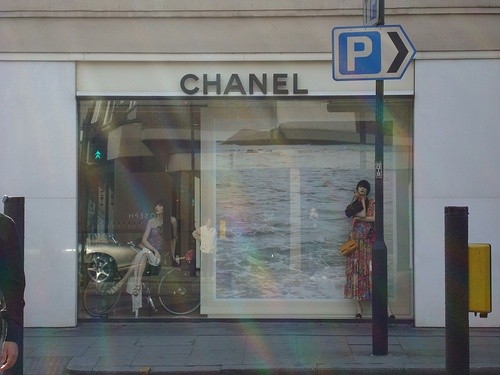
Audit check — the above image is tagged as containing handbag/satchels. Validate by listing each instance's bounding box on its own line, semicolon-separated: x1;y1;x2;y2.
344;195;364;218
340;239;357;256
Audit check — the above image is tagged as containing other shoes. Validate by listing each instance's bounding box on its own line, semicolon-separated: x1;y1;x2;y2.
389;314;395;319
105;284;121;296
355;313;362;320
133;285;140;296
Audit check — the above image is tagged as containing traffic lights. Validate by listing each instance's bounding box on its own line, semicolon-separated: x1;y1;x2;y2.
88;132;108;163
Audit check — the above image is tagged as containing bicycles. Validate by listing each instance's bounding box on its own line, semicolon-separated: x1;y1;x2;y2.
84;255;201;316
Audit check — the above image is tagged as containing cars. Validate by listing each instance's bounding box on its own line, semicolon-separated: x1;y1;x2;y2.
78;233;161;282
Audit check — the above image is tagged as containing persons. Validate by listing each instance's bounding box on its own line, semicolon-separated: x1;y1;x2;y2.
192;217;218;282
344;180;396;320
106;199;186;297
0;212;25;375
